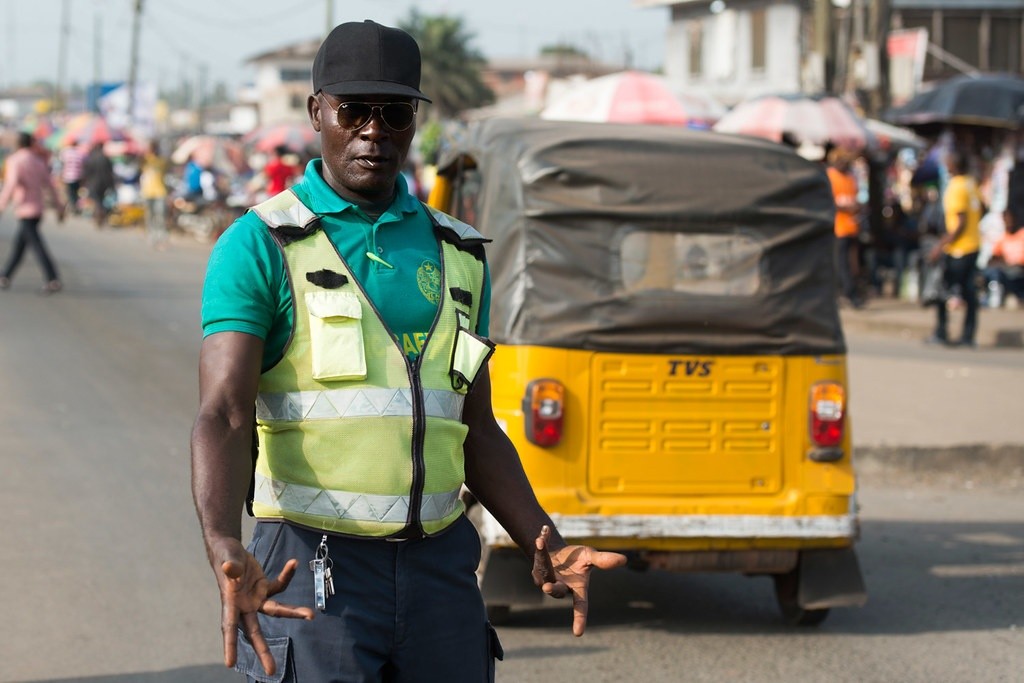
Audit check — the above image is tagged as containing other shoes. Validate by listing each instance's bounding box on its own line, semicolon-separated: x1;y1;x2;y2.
39;280;60;293
0;277;11;290
925;335;944;345
957;337;978;351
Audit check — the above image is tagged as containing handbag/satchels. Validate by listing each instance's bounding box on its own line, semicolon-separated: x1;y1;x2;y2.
920;258;948;307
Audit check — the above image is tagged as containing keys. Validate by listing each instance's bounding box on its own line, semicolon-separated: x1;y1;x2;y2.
321;567;341;598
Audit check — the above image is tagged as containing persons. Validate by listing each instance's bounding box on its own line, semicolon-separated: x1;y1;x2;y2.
1;126;436;244
765;128;1024;349
0;131;67;296
191;17;628;683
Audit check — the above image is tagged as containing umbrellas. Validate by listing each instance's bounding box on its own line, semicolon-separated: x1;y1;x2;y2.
543;72;1024;133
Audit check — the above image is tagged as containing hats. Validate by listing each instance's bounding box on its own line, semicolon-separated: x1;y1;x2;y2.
313;19;433;105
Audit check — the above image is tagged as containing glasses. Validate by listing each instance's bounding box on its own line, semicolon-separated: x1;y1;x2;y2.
319;90;419;131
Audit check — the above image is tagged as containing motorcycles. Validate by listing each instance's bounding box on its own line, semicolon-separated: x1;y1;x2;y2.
427;122;868;628
